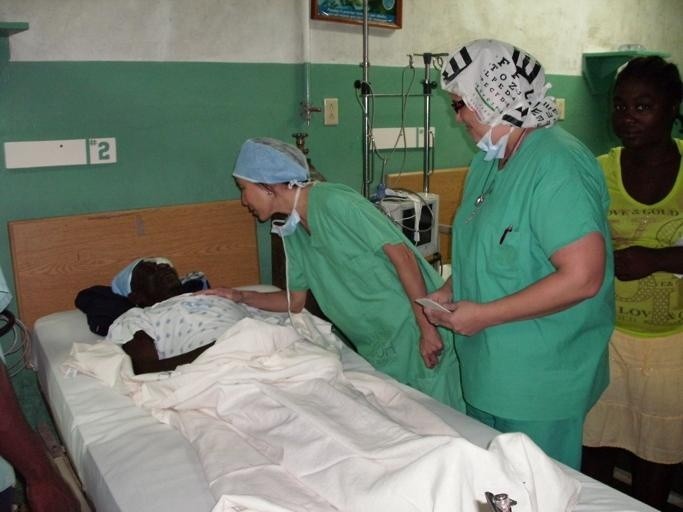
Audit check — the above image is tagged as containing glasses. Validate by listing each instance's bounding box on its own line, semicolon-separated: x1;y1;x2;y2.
451;100;465;114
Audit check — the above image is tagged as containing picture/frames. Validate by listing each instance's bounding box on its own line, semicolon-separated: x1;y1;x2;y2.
311;0;403;29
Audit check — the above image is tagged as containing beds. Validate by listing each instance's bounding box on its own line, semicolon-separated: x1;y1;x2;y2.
7;199;663;512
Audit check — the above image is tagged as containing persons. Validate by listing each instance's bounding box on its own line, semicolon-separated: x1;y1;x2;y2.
194;136;467;416
111;254;254;374
0;347;81;512
420;39;616;470
581;56;683;509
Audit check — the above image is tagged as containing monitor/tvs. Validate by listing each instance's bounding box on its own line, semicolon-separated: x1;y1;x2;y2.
374;193;440;258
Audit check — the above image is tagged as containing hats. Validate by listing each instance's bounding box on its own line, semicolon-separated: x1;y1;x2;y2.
112;257;145;298
232;136;310;183
441;37;561;129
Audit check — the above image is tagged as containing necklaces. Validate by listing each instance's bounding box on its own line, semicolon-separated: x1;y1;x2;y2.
476;127;529;206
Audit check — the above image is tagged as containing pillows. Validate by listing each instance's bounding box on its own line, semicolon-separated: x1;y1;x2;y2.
73;276;212;337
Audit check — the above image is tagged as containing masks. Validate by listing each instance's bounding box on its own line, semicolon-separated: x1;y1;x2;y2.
272;186;301;239
476;119;514;162
